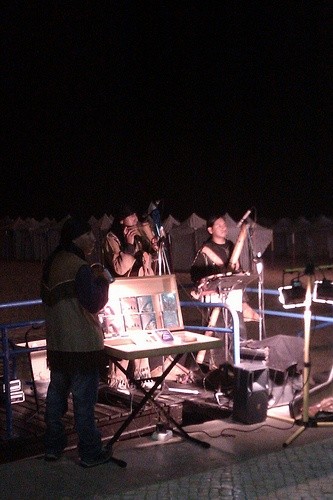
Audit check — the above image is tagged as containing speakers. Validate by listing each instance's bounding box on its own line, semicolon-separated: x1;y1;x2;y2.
233;363;268;425
271;221;333;269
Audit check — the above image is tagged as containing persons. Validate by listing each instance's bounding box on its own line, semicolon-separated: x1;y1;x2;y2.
105;203;158;278
41;215;115;466
190;216;246;362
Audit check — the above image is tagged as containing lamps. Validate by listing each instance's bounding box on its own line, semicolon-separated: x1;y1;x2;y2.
311;279;333;304
278;281;308;312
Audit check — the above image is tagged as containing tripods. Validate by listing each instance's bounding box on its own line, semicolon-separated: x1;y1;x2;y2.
265;269;333;449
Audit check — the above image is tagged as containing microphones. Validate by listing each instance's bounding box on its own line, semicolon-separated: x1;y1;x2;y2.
237;209;252;227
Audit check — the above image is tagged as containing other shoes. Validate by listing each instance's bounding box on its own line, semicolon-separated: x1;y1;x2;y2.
81;448;114;468
45;449;62;459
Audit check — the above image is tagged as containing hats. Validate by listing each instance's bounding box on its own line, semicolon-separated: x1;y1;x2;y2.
60;218;92;243
113;205;136;223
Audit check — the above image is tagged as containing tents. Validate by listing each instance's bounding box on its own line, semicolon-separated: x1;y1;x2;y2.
161;207;333;273
0;214;113;262
